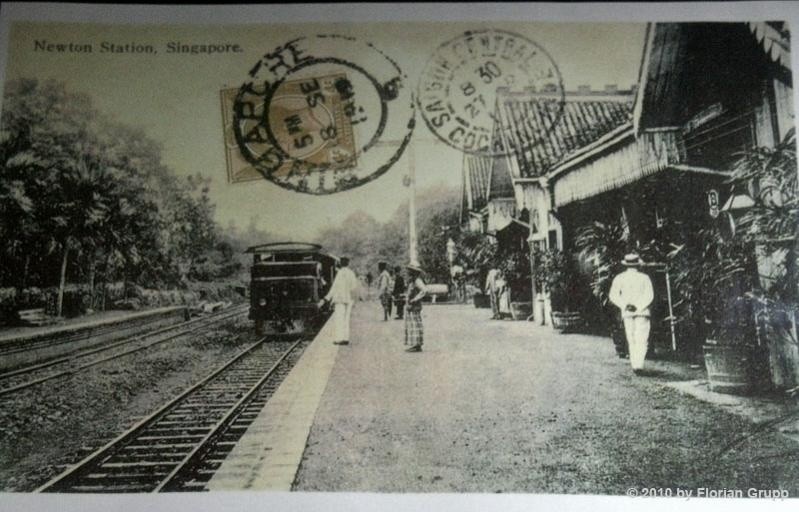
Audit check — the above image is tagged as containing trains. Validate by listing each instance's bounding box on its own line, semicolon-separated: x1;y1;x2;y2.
243;240;344;338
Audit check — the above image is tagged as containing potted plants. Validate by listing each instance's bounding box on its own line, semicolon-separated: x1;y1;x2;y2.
536;247;582;334
452;229;493;309
673;247;764;395
502;257;534;323
578;221;634;359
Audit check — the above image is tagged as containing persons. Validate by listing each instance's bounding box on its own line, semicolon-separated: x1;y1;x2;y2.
498;264;512;314
377;261;393;323
485;263;500;320
609;254;655;376
403;261;429;352
318;254;360;345
393;266;405;319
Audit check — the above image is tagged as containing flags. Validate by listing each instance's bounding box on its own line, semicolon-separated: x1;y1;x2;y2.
700;173;719;219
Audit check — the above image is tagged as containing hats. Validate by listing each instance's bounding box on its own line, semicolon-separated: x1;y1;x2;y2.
620;253;644;266
399;260;426;276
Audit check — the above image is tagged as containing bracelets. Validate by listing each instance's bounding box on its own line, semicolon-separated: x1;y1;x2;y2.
321;297;328;304
